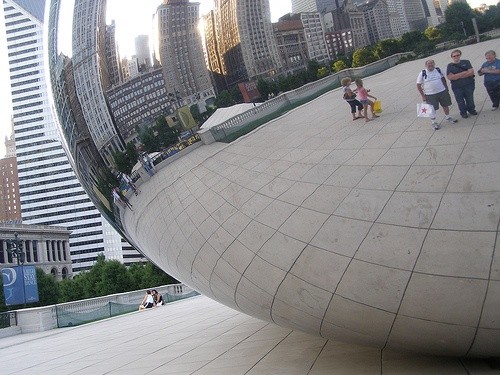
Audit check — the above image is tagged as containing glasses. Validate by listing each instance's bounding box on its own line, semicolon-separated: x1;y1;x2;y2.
451;54;460;58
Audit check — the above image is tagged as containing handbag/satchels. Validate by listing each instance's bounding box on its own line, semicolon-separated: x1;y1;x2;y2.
343;86;357;99
372;99;384;113
417;100;436;118
157;300;162;306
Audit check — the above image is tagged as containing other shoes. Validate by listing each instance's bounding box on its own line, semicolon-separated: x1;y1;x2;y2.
469;109;477;115
445;117;458;123
461;111;468;118
491;106;497;110
431;122;439;131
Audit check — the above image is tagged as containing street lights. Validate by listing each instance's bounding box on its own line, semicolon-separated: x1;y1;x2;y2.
6;232;24;265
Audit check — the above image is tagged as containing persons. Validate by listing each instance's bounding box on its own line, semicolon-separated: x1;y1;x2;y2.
341;76;380;122
112;153;155;211
137;289;165;311
416;49;500;130
